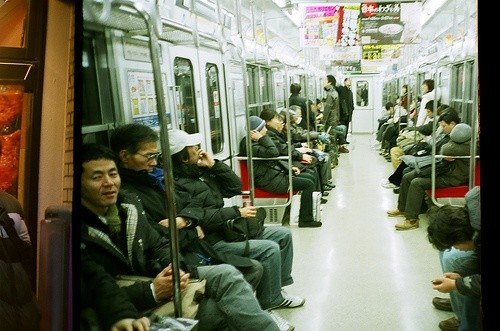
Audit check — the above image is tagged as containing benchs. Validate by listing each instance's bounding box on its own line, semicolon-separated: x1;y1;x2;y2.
236;155;297;225
424;156;480;207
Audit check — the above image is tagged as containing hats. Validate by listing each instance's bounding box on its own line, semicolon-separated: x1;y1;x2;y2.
248;116;265;133
158;129;202;157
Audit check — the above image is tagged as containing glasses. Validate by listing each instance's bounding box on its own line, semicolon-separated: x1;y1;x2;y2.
125;150;161;162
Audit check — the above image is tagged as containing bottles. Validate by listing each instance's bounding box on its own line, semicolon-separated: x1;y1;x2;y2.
417;150;428;155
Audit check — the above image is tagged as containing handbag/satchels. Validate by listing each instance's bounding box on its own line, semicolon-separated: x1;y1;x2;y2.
404;140;430;158
223;207;266;242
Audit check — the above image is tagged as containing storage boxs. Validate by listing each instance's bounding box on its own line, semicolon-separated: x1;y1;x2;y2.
289;190;322;228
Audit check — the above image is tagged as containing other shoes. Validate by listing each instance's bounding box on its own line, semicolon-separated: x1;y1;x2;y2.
376;144;391;162
387;208;405;217
439;316;462;331
344;141;350;144
382;181;396;188
320;163;336;203
432;297;453;311
298;219;321;227
339;146;349;153
394;219;419;229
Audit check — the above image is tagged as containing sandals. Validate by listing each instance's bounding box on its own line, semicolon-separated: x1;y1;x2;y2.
394;187;401;193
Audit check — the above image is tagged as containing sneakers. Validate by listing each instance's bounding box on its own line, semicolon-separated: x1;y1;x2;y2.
270;289;304;308
261;307;294;331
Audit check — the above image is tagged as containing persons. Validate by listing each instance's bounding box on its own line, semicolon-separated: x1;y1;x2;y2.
238;74;355;228
0;124;305;330
356;81;368;107
376;78;481;331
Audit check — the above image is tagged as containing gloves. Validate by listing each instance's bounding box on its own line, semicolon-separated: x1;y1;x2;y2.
319;132;330;144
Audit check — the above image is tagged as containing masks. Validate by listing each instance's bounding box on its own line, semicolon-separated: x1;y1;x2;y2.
295;116;302;125
